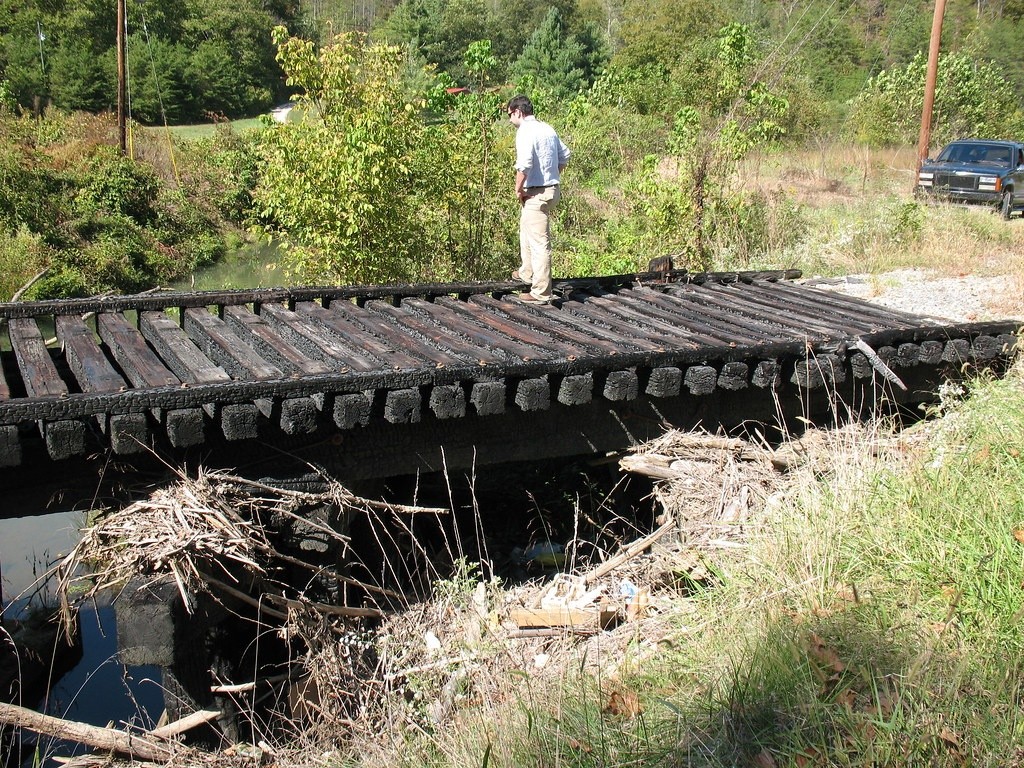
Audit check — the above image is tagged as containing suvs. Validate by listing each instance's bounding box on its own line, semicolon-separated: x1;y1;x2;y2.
916;139;1024;221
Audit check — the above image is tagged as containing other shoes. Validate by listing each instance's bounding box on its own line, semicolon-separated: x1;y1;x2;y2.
519;293;548;305
512;270;532;285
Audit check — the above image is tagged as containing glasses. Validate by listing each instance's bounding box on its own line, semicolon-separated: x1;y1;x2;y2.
508;109;516;118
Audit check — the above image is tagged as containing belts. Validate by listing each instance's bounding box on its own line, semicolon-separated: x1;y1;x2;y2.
523;183;558;189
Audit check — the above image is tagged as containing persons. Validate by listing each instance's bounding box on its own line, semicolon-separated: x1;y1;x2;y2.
506;94;572;305
